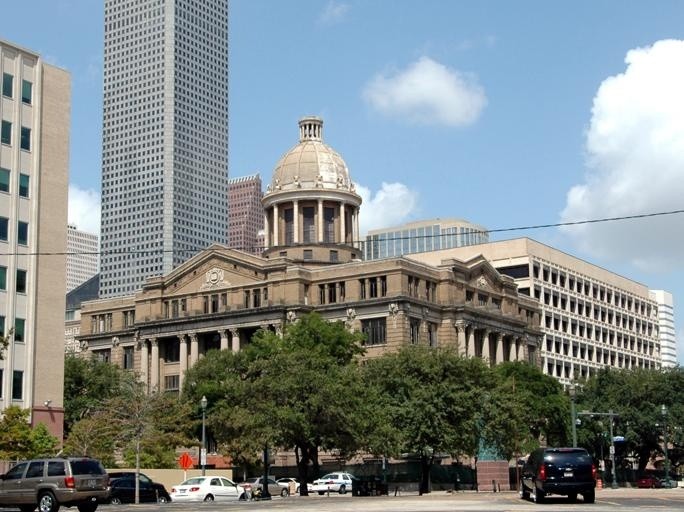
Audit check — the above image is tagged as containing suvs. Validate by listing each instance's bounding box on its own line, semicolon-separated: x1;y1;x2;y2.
1;454;113;512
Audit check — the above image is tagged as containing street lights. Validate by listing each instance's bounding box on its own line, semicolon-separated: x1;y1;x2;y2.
568;384;581;448
660;405;671;488
200;395;207;475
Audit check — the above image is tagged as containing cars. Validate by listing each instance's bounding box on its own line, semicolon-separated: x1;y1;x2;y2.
659;475;678;488
636;474;662;488
238;477;290;499
276;477;315;493
98;471;173;504
169;476;247;503
311;471;358;495
516;447;595;505
